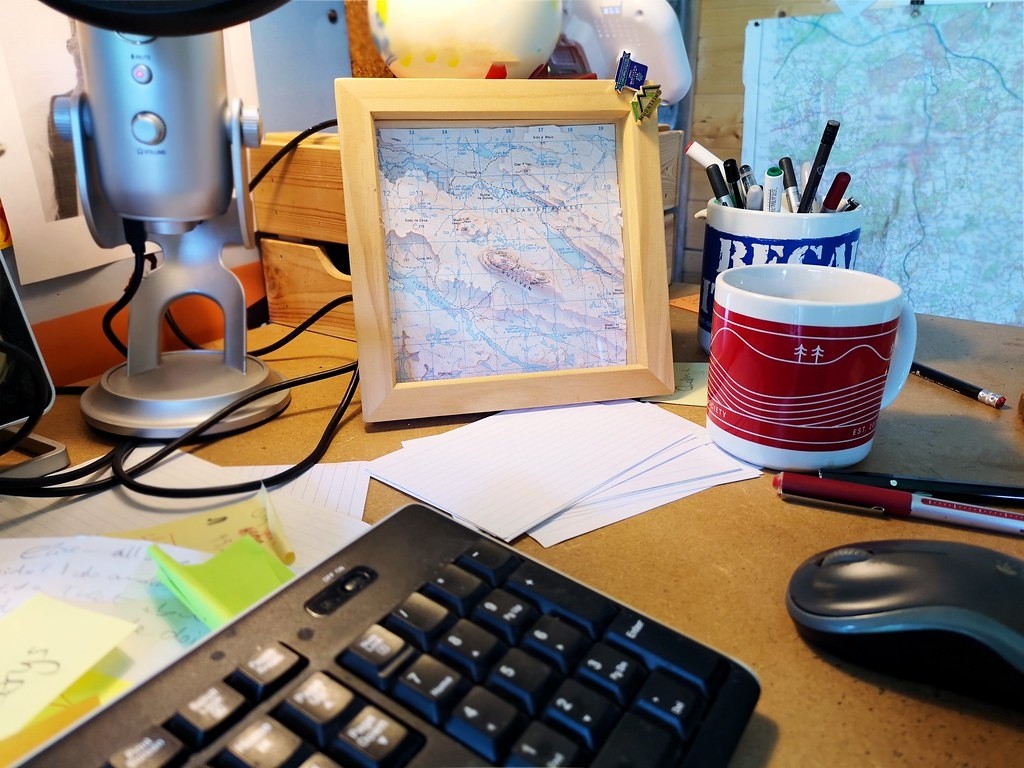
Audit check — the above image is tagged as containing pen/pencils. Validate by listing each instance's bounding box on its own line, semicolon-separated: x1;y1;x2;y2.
771;472;1024;537
683;120;859;213
817;467;1024;508
909;361;1006;410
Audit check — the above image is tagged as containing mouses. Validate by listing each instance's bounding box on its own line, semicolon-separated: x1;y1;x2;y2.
786;540;1024;729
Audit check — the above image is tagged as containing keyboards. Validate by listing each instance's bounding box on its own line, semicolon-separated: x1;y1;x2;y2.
13;502;762;768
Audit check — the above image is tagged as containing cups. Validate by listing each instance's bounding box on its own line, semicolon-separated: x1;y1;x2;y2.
706;264;917;473
697;195;864;356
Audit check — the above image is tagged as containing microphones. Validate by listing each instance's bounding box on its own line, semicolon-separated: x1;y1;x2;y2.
38;0;289;237
